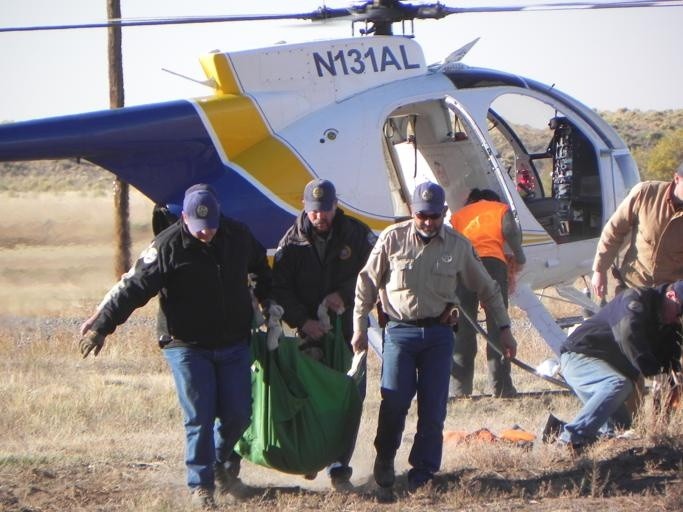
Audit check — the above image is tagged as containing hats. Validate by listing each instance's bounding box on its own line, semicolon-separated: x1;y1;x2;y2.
303;180;334;214
184;182;220;235
673;279;683;302
412;184;444;212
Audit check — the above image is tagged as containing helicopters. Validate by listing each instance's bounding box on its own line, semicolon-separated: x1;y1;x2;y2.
0;0;683;363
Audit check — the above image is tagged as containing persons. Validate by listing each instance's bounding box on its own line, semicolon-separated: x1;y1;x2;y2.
591;164;681;309
539;279;682;467
75;180;287;509
350;181;517;502
270;172;389;491
447;184;528;397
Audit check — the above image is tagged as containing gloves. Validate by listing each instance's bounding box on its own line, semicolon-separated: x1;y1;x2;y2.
79;330;106;360
258;298;277;317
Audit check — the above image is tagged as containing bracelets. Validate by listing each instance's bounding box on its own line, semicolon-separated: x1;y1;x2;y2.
495;325;510;333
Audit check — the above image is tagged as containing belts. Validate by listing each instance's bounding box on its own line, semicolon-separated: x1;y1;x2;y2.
407;317;437;325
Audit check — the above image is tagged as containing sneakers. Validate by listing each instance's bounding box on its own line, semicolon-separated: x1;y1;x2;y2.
191;485;214;511
408;469;432;496
376;451;396;490
536;413;560;447
491;384;518;398
332;478;354;493
213;467;249;502
